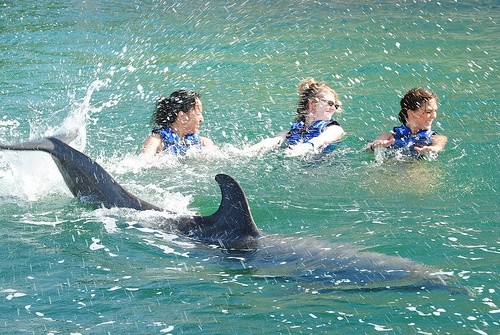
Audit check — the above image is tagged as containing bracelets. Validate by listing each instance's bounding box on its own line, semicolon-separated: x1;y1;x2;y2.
370;144;375;151
304;141;315;151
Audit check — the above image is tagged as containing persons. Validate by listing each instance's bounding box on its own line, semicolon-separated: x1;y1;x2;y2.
364;86;448;160
138;88;218;158
246;74;347;159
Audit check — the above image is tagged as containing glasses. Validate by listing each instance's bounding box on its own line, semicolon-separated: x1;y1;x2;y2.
322;100;340;109
